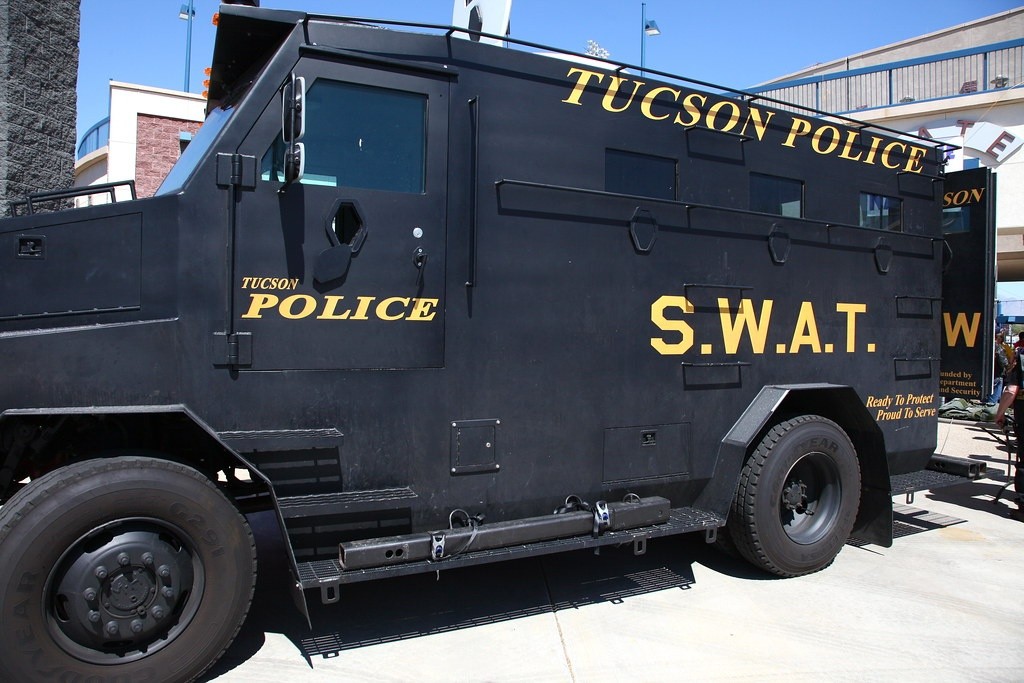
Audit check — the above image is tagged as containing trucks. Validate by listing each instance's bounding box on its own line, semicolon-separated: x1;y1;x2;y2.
0;7;996;683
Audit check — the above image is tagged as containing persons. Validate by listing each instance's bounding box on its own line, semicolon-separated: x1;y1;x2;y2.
994;328;1024;513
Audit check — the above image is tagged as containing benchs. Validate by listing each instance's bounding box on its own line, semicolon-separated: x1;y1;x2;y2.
974;414;1018;506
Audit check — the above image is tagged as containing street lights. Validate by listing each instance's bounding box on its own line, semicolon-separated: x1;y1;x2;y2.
641;3;660;76
179;1;195;93
